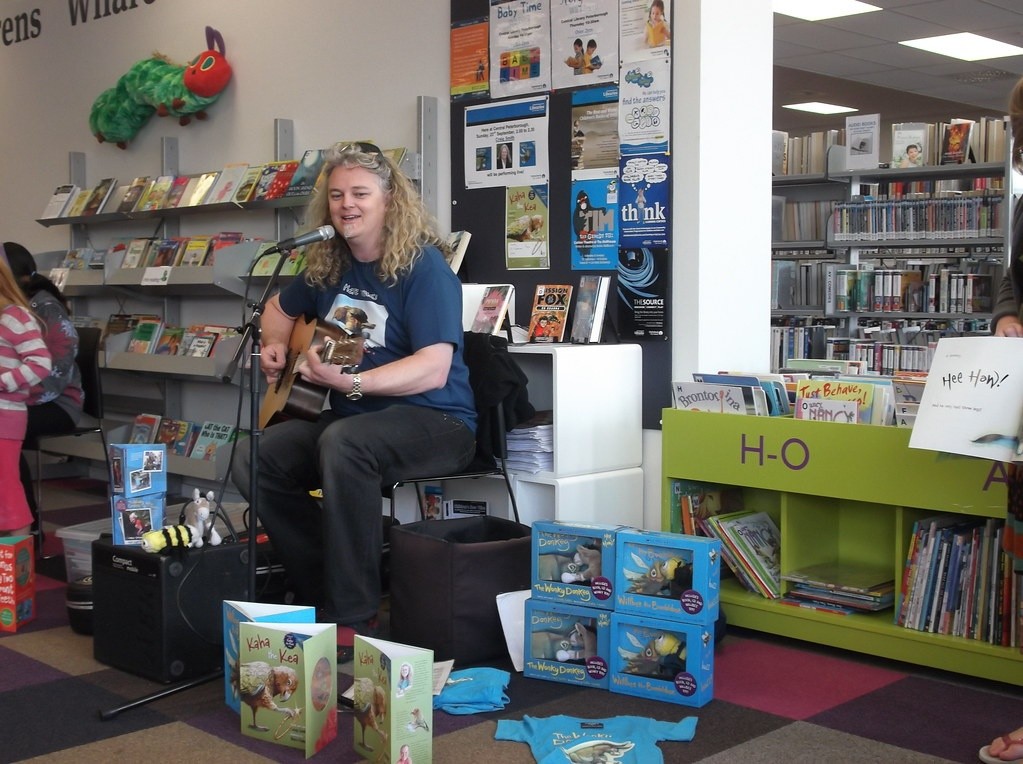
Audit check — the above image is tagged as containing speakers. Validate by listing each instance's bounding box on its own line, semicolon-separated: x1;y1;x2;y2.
89;536;254;685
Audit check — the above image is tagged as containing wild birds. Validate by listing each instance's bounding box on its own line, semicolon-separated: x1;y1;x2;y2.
354;677;387;752
240;661;303;732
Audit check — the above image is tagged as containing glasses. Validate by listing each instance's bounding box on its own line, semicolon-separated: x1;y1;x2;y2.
339;142;384;160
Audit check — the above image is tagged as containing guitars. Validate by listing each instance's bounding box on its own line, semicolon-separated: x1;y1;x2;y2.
258;311;366;431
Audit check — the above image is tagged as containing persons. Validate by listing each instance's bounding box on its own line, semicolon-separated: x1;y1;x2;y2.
497;143;513;169
564;38;605;76
231;142;478;664
897;144;922;169
979;74;1023;764
573;119;585;140
0;242;86;546
641;0;671;49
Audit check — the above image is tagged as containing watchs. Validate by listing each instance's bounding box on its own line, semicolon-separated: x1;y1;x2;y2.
347;372;363;401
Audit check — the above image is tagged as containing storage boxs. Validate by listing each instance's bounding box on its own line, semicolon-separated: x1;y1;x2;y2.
55;500;252;589
390;514;720;711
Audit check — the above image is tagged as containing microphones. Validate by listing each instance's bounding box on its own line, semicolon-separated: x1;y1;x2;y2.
262;224;336;255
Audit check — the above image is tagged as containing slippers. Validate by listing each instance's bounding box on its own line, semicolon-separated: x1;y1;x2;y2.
978;734;1023;764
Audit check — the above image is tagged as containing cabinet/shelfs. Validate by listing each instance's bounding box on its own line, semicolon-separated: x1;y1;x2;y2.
771;120;1020;360
404;337;650;525
32;194;312;483
661;407;1023;692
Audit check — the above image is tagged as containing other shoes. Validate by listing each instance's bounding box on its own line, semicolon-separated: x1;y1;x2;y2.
334;615;381;665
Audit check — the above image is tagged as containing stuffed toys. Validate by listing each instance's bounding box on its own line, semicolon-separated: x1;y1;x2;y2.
88;26;232;150
142;488;220;554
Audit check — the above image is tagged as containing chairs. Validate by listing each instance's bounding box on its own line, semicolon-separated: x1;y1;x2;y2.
382;331;541;525
23;324;113;560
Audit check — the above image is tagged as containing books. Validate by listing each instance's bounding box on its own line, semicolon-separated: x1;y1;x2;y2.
670;479;1023;648
47;231;346;279
773;112;1009;379
62;312;247;367
130;414;251;468
41;148;412;220
471;275;619;344
671;336;1023;464
